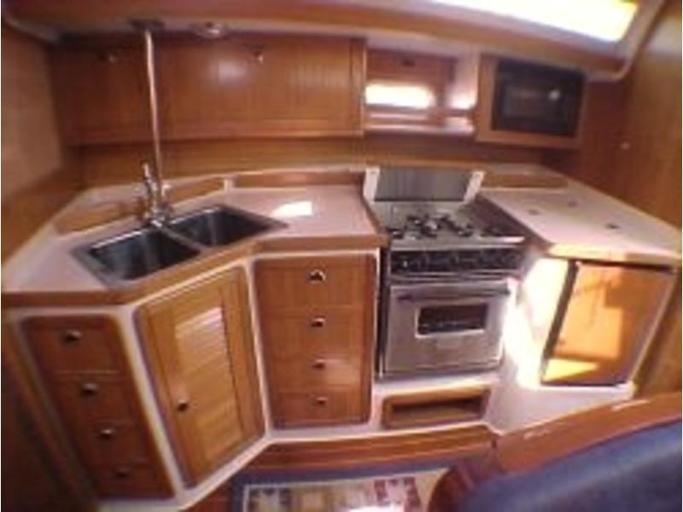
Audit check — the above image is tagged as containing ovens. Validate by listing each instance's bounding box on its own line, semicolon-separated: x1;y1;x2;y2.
378;275;518;381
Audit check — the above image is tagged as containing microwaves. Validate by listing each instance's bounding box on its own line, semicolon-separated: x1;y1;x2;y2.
488;55;587;139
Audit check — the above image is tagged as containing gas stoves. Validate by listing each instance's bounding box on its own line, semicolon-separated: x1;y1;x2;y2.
373;197;528;281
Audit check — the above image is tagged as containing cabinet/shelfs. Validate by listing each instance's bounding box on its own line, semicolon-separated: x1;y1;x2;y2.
131;261;273;507
474;50;590;149
251;244;382;443
49;33;367;147
542;257;676;388
2;306;185;511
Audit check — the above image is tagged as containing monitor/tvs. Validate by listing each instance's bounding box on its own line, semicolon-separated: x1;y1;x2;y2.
491;62;583;138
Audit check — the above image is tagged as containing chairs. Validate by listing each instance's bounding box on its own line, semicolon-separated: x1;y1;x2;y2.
425;390;681;509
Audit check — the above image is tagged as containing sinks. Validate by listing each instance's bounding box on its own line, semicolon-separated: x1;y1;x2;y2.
165;204;286;250
69;225;202;288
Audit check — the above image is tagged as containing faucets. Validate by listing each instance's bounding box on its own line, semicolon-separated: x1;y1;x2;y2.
139;163;159;221
